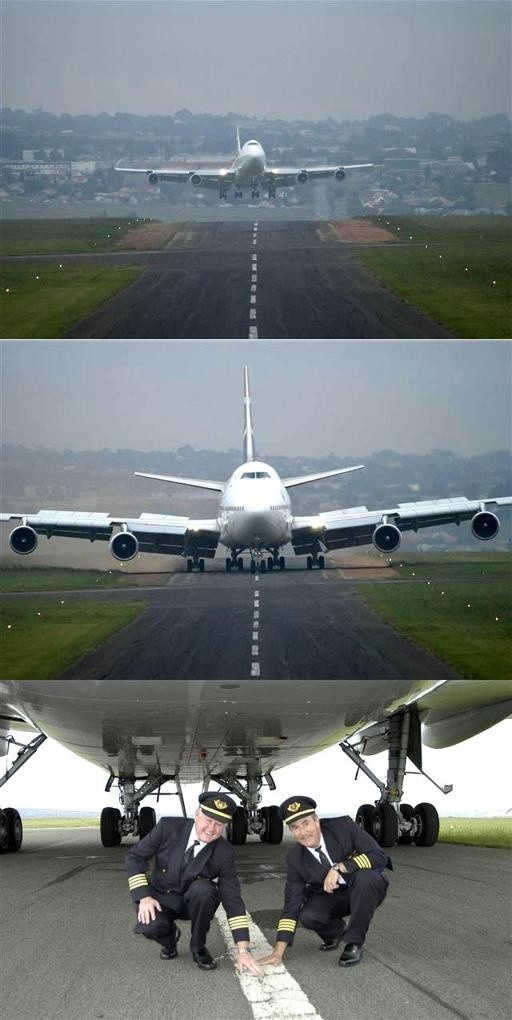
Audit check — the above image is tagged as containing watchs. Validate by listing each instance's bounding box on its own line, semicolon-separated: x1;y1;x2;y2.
332;863;343;875
238;948;251;954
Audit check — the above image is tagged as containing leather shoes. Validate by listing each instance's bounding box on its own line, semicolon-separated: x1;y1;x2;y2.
318;924;347;952
192;943;216;970
338;943;364;968
159;926;181;960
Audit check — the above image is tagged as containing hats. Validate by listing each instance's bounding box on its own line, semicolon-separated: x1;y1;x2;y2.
276;795;317;825
197;790;238;824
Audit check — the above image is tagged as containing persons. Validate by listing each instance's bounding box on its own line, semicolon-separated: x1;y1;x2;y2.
255;795;395;967
126;791;266;976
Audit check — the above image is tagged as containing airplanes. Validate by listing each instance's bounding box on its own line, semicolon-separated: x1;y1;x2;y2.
0;367;511;570
0;678;511;858
115;123;372;204
